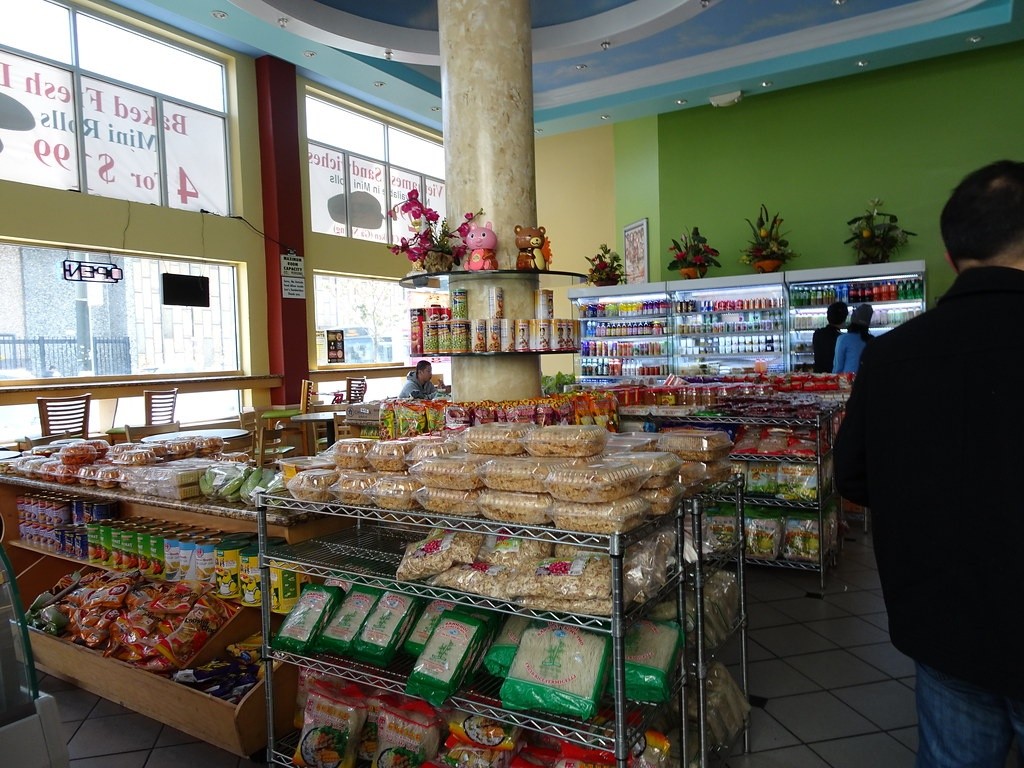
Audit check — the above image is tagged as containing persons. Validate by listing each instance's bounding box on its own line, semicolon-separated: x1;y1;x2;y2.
398;361;444;400
832;304;873;373
832;159;1024;768
812;302;849;374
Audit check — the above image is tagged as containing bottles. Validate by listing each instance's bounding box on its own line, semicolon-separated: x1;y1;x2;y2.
580;277;922;378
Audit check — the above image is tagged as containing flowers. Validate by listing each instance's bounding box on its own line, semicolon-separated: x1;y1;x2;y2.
737;203;800;265
666;224;722;278
843;198;918;262
585;243;631;285
386;188;484;271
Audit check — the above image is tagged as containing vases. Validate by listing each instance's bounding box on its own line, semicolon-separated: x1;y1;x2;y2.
679;267;707;279
593;278;619;286
753;259;784;272
423;251;454;272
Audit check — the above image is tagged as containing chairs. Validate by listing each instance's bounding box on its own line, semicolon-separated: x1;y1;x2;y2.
13;374;367;468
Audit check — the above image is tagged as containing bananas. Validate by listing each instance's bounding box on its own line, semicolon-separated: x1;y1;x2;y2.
198;465;289;508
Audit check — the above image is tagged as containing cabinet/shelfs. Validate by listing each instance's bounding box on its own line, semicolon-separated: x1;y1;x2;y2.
398;268;588;357
0;398;852;768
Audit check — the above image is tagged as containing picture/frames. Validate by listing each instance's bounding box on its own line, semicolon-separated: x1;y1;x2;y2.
622;218;650;283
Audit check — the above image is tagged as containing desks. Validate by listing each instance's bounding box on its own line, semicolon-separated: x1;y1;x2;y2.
289;410;348;449
0;374;285;406
140;428;252;441
308;366;417;381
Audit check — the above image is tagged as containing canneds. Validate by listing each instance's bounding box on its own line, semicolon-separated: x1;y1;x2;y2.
421;287;582;355
16;490;299;614
588;340;669;356
676;297;780;313
643;383;769;406
639;365;668;375
700;336;719;354
678;318;783;334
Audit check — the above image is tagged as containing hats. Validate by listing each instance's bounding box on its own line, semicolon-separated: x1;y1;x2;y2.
851;304;874;326
